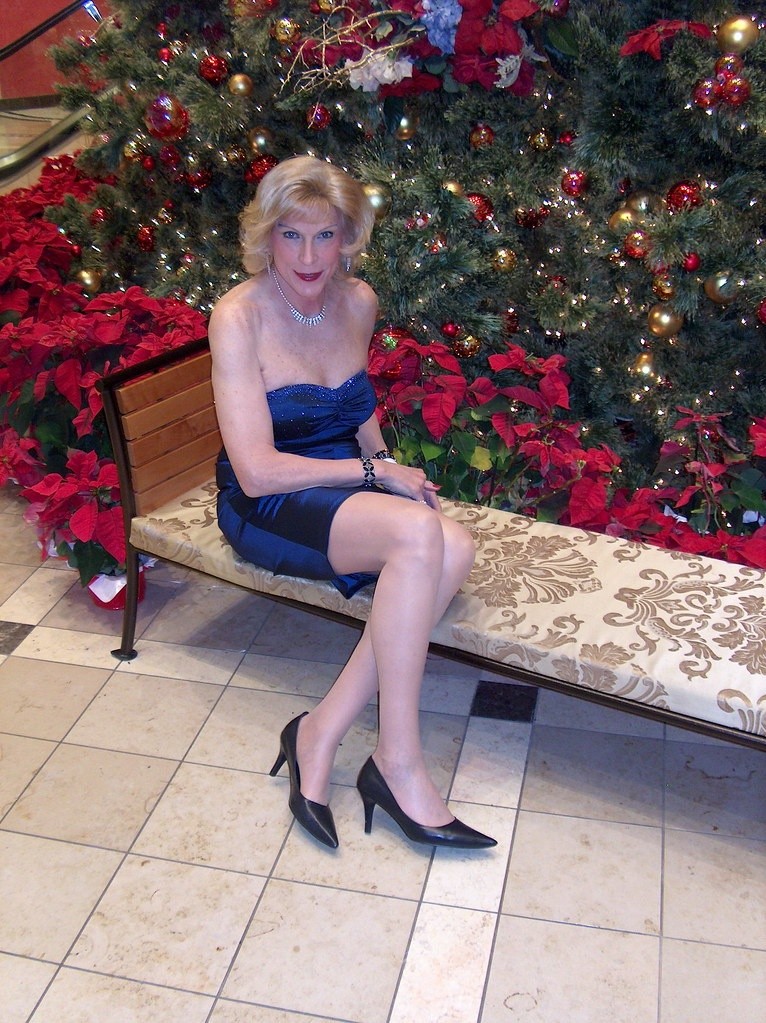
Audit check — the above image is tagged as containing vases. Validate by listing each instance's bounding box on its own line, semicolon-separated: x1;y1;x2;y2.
88;570;146;610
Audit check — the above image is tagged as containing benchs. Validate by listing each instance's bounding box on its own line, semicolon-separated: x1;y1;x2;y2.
95;336;766;752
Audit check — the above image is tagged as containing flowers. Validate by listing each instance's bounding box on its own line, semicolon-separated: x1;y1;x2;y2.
0;0;766;589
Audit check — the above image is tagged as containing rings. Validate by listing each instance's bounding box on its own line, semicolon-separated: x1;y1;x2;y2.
420;499;426;504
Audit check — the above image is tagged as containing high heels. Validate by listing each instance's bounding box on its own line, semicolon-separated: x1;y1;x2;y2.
269;711;339;849
357;756;497;848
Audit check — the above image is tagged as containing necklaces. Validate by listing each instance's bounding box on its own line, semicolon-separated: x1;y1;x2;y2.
272;268;326;327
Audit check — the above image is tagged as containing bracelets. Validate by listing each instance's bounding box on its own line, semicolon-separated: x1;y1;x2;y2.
362;457;376;486
371;449;397;463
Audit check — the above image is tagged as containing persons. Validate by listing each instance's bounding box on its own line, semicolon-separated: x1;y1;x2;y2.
210;156;499;849
